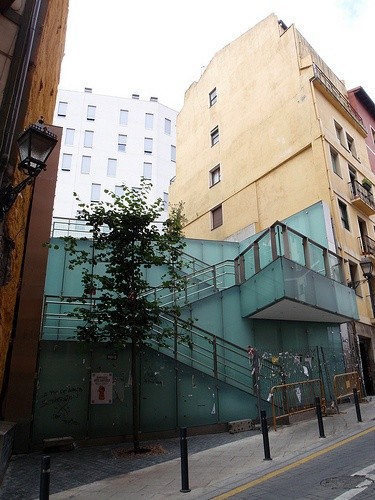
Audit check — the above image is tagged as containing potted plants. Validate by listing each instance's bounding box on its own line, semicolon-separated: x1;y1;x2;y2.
362;178;373;191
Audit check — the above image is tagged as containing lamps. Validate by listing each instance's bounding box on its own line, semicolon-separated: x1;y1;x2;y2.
348;254;375;290
1;114;59;225
